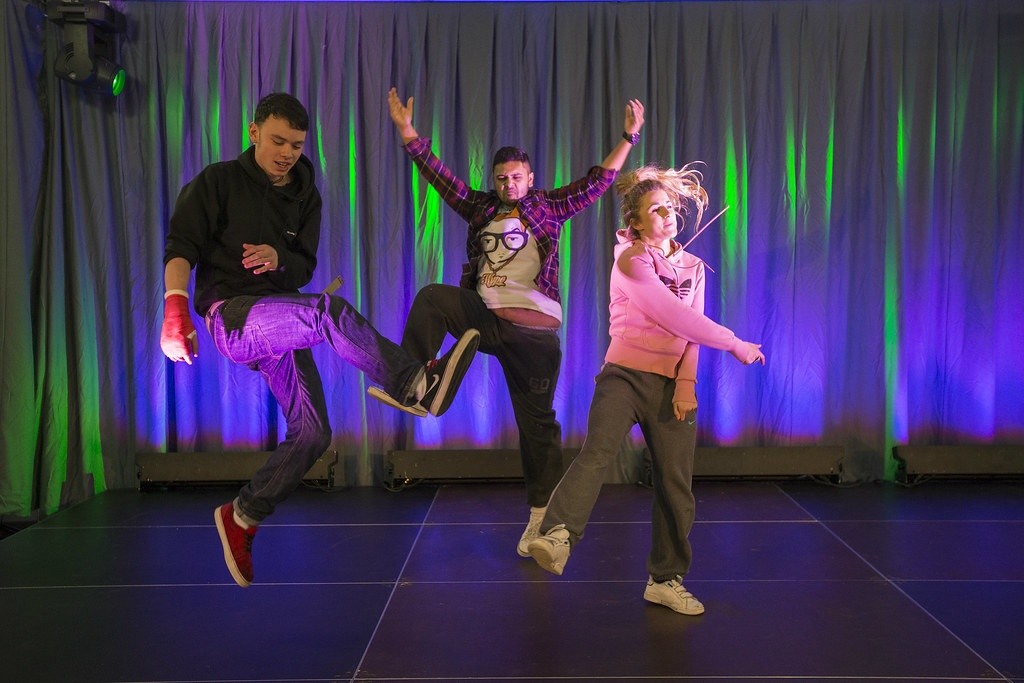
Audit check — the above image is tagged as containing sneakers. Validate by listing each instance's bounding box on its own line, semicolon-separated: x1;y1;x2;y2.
518;506;547;557
643;574;705;615
214;503;253;587
529;523;569;575
368;385;427;417
420;329;480;418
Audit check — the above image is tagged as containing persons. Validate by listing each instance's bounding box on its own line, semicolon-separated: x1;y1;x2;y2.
158;92;483;592
522;159;767;618
367;85;646;559
